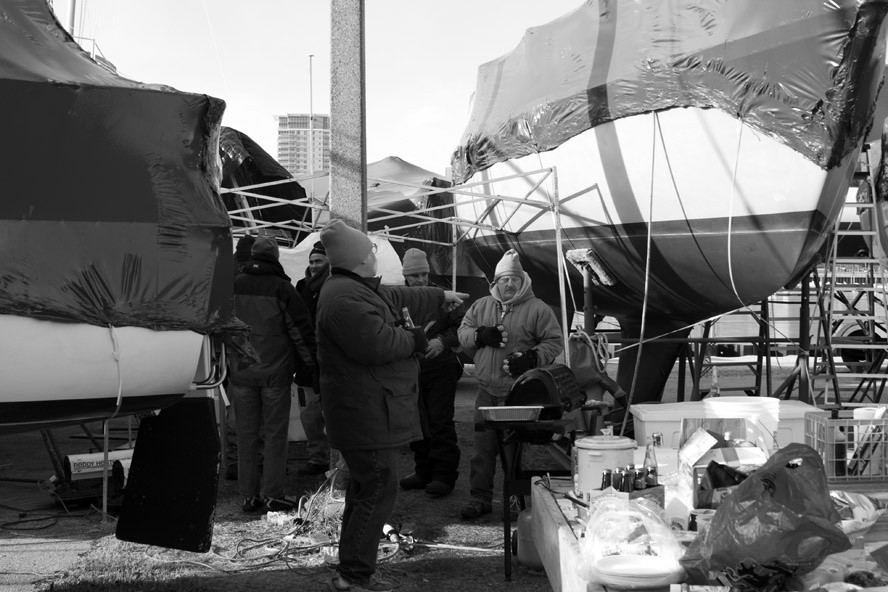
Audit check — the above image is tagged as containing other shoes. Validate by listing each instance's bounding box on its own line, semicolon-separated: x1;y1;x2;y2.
327;572;393;592
261;499;293;513
426;482;450;494
243;497;262;512
298;464;330;476
400;474;426;487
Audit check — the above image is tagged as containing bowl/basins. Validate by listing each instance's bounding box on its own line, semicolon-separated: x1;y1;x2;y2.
591;555;685;589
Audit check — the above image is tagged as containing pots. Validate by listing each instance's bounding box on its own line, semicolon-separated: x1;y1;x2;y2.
573;428;636;495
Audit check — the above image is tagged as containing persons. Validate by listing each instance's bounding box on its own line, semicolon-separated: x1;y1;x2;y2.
456;249;564;522
213;235;315;515
315;220;469;592
295;241;332;474
400;248;467;499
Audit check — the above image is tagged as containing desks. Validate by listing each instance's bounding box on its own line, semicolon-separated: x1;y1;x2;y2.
474;420;577;582
531;476;613;592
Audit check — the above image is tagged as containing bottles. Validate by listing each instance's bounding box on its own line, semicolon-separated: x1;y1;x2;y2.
600;438;659;492
382;523;399;543
710;366;720;398
401;306;415;328
829;409;847;476
571;429;585;484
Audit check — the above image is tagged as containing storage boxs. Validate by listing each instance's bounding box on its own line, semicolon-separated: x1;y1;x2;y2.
630;400;888;493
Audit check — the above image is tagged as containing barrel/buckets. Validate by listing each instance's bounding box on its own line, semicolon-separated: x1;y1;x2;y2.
704;395;779;451
853;407;888;475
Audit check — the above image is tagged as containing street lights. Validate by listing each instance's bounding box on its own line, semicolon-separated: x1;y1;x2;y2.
308;53;315;203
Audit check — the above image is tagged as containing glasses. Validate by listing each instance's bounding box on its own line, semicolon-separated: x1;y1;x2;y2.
372;244;377;255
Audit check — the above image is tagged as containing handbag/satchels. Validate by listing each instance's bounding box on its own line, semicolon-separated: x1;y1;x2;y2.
682;444;853;587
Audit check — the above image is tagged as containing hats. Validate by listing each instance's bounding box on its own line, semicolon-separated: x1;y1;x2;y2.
254;231;278;256
494;249;525;279
320;219;374;271
310;241;326;256
401;248;430;274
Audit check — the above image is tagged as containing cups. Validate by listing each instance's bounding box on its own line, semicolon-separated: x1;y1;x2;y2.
689;509;716;534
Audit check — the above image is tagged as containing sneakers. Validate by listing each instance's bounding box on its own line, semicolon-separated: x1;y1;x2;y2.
502;496;523;521
461;501;493;519
